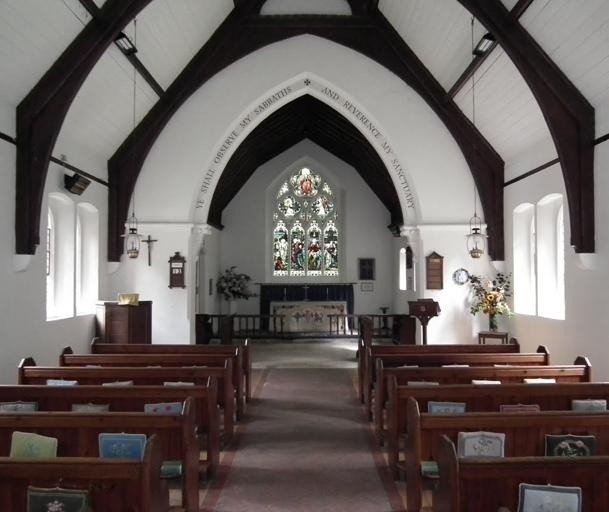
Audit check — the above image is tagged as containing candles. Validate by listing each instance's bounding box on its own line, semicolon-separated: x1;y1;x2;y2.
327;288;329;296
285;288;286;296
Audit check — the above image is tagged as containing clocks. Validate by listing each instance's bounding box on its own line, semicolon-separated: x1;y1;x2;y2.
453;269;469;284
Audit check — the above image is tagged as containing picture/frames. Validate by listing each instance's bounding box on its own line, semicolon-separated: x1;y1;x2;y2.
358;280;375;294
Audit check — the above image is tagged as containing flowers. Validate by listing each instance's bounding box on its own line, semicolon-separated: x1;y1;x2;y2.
216;267;260;301
467;273;517;318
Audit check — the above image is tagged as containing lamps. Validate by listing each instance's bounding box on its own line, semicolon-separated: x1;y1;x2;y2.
465;16;495;259
113;13;143;259
64;174;90;196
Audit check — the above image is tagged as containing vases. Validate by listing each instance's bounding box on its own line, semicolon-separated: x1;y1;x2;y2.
228;299;239;331
489;312;498;331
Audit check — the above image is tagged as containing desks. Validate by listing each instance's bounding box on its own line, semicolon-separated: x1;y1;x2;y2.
267;300;349;333
479;331;508;344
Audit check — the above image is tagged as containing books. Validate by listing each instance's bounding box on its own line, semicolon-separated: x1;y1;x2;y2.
7;429;60;458
457;430;506;458
426;399;467;412
543;430;601;457
499;402;541;412
1;399;40;411
572;399;608;410
25;482;93;512
97;429;148;460
70;400;110;412
144;399;182;414
514;481;582;511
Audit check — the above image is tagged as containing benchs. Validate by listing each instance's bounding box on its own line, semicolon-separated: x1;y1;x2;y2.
0;339;252;512
356;339;608;512
